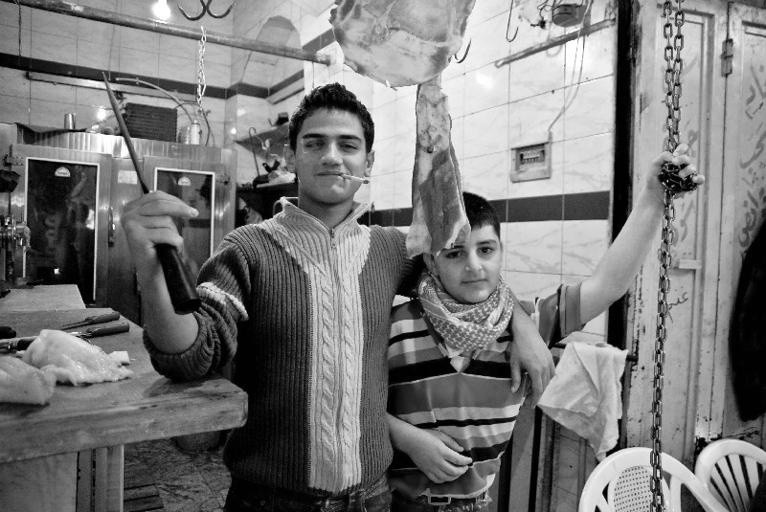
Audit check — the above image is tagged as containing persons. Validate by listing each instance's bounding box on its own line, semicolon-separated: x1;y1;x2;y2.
379;141;706;511
119;82;422;510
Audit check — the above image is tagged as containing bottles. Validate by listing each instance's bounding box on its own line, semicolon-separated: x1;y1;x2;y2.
181;120;200;145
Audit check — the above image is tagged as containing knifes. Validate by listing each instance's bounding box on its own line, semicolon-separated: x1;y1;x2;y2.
57;313;130;340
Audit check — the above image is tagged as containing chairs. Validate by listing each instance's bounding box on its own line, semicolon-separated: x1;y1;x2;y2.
578;439;766;512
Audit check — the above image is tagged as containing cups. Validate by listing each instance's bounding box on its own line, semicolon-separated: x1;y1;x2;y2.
64;112;77;130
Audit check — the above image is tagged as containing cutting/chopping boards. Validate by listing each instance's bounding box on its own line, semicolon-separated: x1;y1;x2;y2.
0;307;249;463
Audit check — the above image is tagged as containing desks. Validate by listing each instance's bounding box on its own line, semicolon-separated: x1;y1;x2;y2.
0;307;251;511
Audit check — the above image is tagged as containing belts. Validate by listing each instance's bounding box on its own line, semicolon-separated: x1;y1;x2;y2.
419;492;485;506
